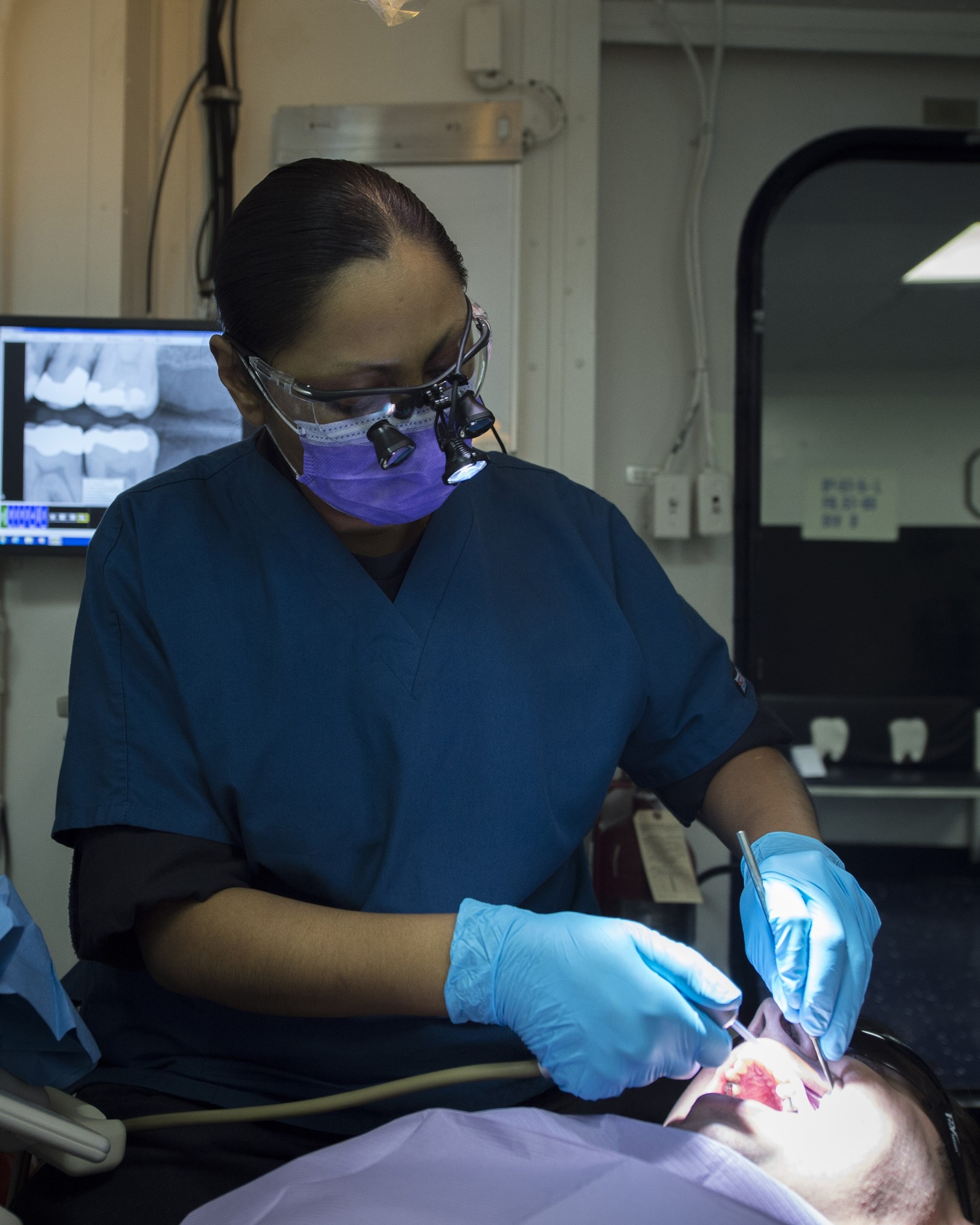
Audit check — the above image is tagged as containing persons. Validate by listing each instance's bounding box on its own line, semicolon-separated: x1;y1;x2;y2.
55;154;886;1103
174;999;978;1222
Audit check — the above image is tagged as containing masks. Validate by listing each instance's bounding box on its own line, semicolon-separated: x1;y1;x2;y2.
243;360;484;528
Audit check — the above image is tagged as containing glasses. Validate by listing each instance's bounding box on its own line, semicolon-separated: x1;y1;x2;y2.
220;298;491;443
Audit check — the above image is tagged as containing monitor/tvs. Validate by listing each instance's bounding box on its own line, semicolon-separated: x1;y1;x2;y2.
0;313;251;560
